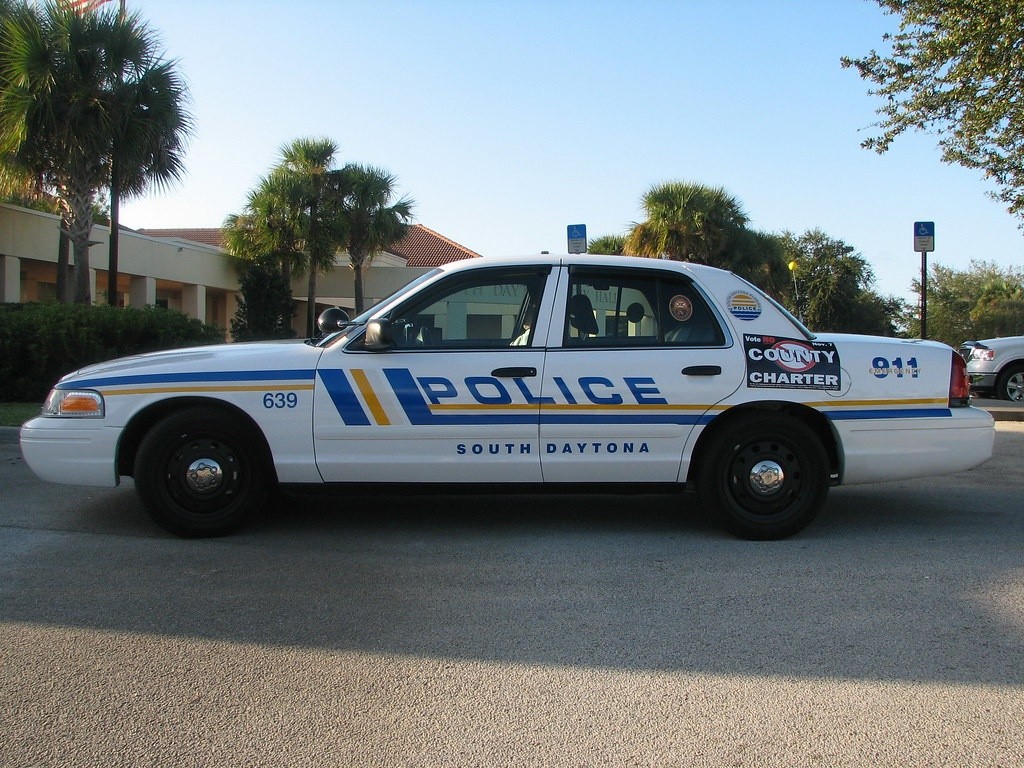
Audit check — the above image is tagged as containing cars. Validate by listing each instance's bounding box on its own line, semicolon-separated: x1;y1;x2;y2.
18;252;995;541
957;335;1024;402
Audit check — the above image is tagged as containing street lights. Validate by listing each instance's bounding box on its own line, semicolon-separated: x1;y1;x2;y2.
789;262;805;324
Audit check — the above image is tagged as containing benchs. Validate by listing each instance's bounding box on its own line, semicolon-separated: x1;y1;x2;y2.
664;318;719;343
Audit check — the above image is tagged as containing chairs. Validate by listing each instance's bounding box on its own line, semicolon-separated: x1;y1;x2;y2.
509;304;536;347
563;296;599;346
